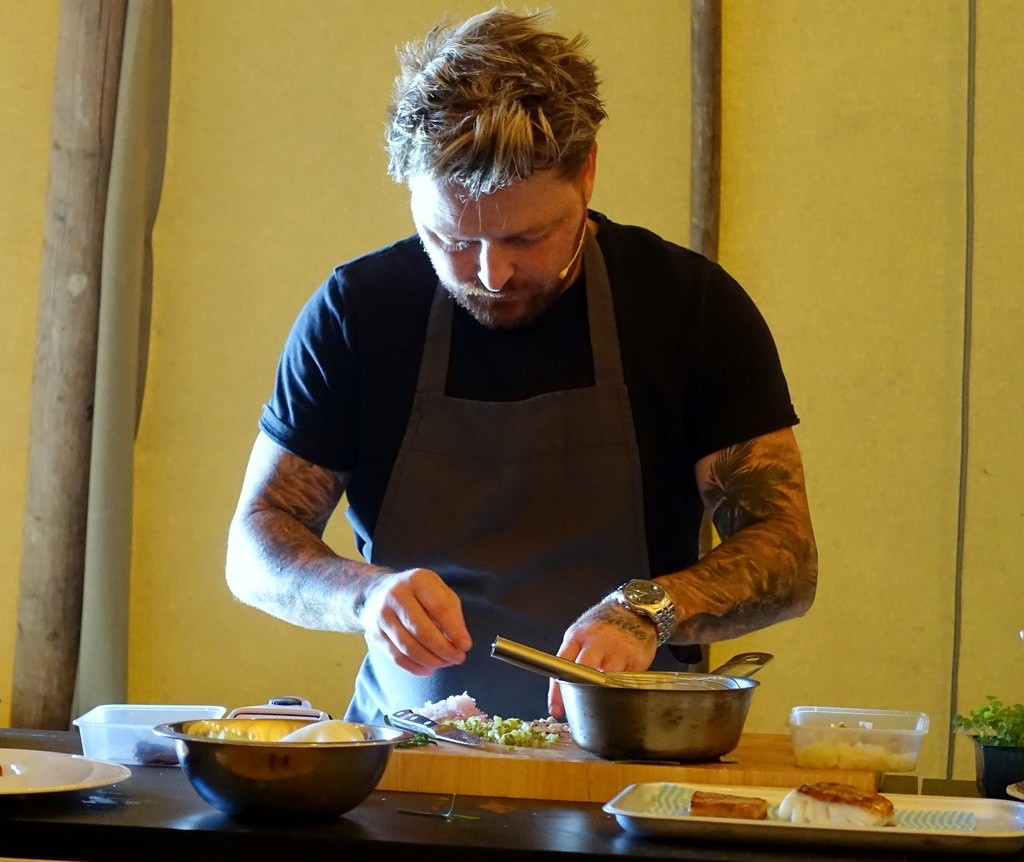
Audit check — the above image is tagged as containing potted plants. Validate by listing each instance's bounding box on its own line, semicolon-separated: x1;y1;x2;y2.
950;692;1024;799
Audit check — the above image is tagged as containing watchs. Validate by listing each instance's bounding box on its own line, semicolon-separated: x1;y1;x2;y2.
600;578;676;647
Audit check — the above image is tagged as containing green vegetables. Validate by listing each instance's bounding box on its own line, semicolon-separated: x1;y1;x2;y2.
951;694;1024;748
384;714;441;750
396;792;482;821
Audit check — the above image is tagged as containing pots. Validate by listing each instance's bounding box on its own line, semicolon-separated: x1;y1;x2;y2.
554;651;774;763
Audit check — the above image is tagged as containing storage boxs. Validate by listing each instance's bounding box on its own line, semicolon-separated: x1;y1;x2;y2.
72;702;226;767
787;705;931;771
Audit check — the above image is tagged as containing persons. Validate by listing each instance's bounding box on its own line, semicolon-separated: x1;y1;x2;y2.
224;7;819;729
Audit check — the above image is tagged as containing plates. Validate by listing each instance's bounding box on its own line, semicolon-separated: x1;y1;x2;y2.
0;747;132;794
1006;781;1024;800
602;781;1024;852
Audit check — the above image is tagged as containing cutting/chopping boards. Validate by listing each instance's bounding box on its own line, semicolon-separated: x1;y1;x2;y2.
376;733;877;803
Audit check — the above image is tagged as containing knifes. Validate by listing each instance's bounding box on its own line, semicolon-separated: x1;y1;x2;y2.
389;709;488;750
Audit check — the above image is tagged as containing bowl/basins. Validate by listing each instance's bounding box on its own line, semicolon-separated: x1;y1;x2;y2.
785;704;930;771
73;704;226;769
151;716;415;820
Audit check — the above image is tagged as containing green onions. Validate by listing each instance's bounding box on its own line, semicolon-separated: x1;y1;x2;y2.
449;713;559;751
